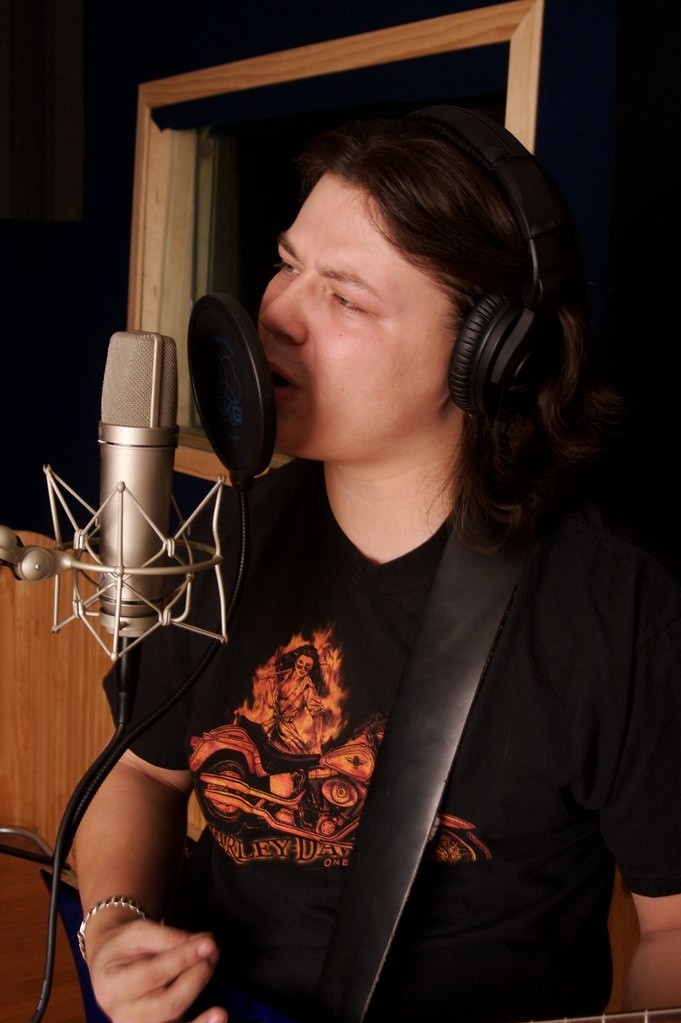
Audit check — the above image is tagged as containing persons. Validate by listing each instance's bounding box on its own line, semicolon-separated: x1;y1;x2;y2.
75;117;680;1023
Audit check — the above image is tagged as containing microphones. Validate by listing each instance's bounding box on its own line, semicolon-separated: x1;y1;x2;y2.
100;329;183;637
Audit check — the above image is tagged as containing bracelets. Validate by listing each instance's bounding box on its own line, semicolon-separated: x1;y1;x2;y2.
76;896;152;962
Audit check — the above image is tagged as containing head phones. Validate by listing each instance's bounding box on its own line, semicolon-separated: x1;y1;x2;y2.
413;105;566;426
